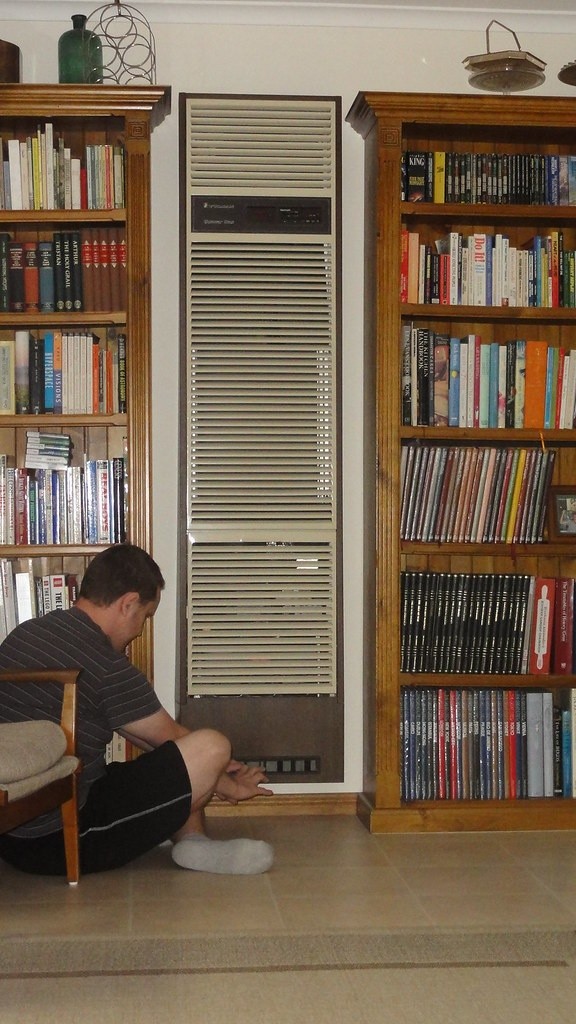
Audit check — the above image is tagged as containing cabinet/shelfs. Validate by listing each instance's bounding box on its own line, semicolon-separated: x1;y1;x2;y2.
343;90;576;832
0;79;172;764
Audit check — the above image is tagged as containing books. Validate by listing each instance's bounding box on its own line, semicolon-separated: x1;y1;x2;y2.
399;445;556;546
400;571;576;677
399;685;575;800
0;327;127;415
0;430;127;545
398;151;576;207
399;222;576;308
400;326;576;430
0;557;80;647
104;729;133;765
0;230;129;313
0;122;125;210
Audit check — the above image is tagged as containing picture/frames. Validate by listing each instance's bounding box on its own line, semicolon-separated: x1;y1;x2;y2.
548;484;576;544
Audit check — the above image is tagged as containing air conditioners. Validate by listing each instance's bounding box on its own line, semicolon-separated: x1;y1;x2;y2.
178;91;343;784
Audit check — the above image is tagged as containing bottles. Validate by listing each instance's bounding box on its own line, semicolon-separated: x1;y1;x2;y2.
57;14;103;84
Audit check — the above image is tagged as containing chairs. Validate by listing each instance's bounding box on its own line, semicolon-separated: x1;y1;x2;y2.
1;668;81;887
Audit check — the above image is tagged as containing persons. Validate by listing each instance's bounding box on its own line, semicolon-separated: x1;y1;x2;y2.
0;546;275;876
559;510;576;533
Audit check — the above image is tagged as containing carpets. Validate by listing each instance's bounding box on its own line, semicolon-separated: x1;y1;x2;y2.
0;923;576;1024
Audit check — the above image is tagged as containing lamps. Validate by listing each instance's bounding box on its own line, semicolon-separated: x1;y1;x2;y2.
463;20;548;95
